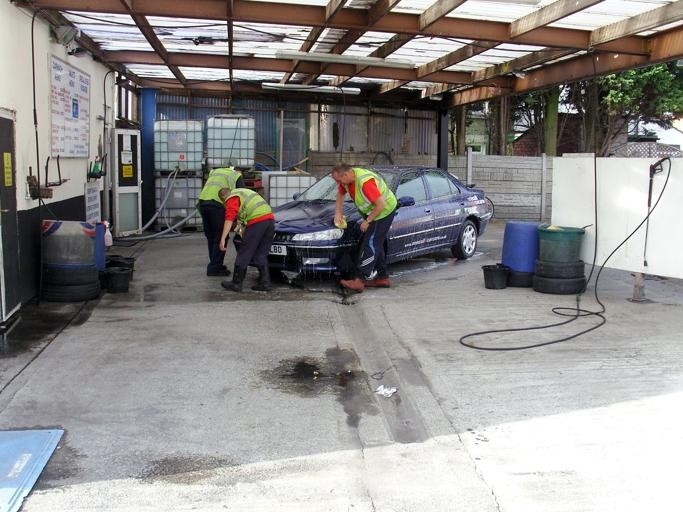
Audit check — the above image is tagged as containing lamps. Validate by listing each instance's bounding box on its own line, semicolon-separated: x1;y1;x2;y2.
47;23;78;49
113;73;130;87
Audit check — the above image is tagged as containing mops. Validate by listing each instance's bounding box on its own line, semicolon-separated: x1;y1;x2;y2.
283;147;314;172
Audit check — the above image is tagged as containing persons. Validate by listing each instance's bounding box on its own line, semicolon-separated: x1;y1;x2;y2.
216;187;275;292
193;165;246;277
330;161;399;293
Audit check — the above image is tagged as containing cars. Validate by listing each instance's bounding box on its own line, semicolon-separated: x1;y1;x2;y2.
231;164;491;281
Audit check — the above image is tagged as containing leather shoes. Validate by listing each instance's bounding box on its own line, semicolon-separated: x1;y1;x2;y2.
364;277;391;289
338;277;365;294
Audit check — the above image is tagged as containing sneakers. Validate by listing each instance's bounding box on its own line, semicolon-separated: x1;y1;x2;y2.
207;264;230;276
218;262;226;269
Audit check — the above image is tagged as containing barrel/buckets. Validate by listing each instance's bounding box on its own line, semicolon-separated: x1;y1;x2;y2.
481;260;510;288
502;219;539;272
538;221;586;262
101;253;136;293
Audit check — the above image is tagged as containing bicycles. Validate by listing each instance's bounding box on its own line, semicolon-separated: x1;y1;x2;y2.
464;183;494;221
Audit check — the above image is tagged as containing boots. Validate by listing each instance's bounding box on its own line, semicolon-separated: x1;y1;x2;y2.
250;264;270;292
217;261;248;293
